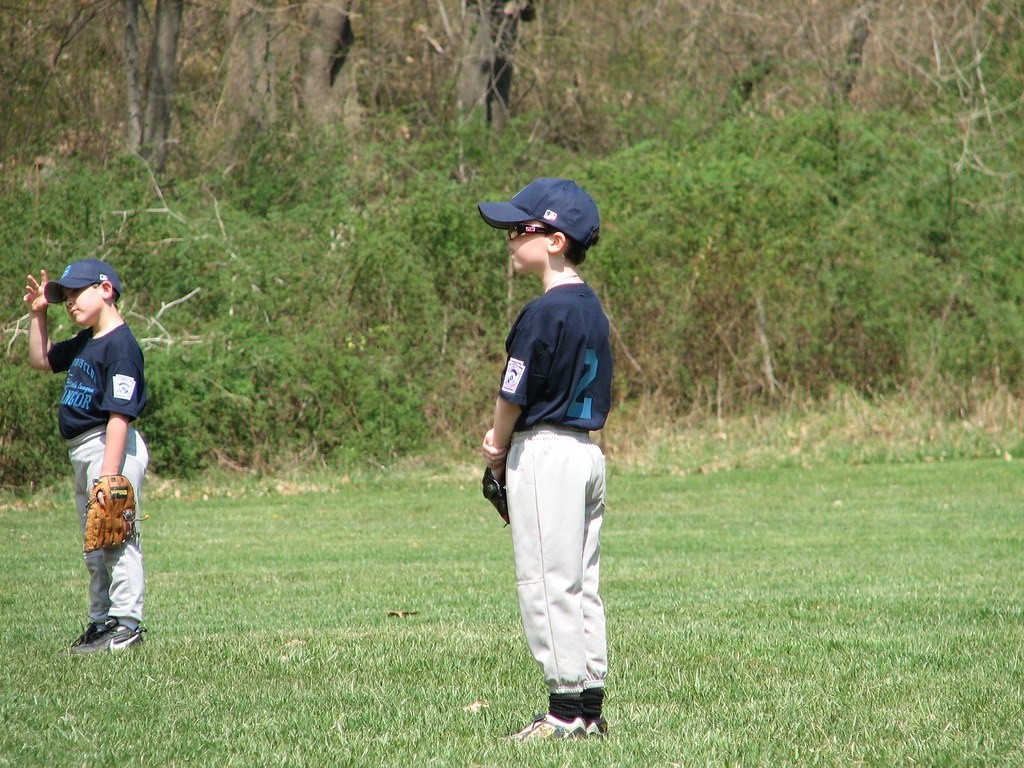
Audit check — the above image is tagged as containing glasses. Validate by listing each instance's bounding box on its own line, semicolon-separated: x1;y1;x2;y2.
505;222;554;241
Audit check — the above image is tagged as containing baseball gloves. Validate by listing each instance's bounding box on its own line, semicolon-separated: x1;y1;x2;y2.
82;474;150;554
481;466;510;528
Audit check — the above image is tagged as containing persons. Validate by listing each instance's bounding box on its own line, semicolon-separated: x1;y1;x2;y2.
478;176;614;744
22;258;149;652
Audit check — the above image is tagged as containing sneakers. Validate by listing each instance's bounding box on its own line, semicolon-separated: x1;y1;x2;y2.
70;616;147;655
501;713;588;742
586;716;608;740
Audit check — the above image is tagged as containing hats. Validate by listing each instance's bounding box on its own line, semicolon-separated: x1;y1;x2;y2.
44;259;121;304
478;178;600;251
